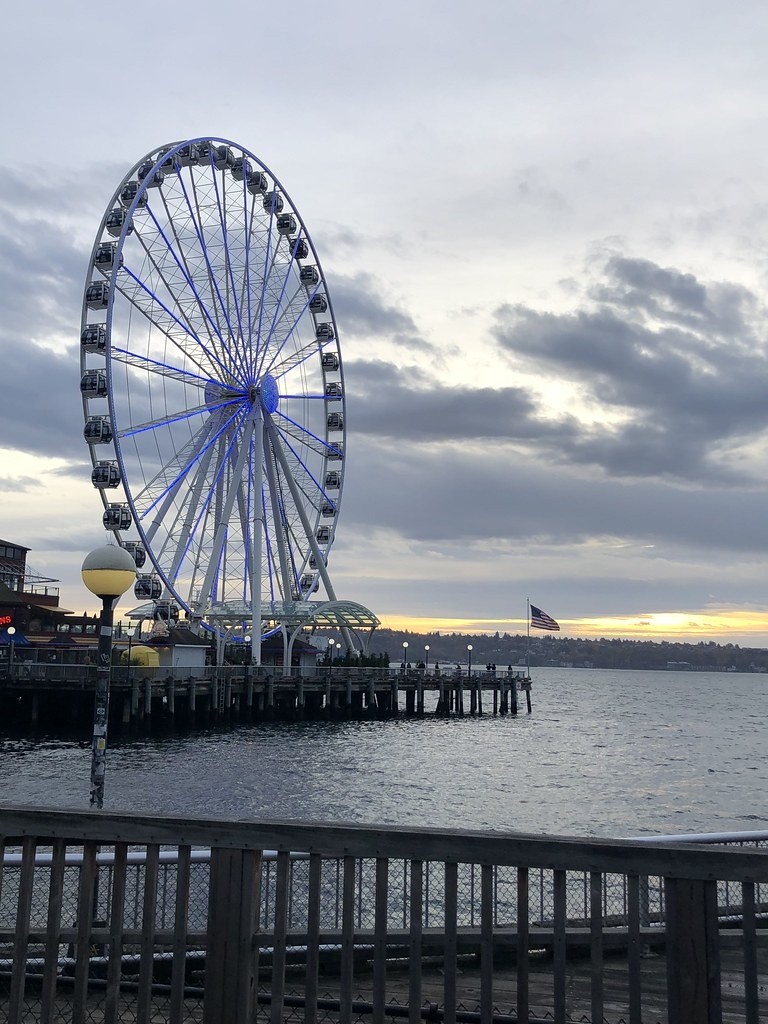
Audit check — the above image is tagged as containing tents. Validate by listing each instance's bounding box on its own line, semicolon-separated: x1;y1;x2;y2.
121;645;160;667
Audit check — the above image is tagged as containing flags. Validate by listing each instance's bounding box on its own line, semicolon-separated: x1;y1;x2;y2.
530;604;560;631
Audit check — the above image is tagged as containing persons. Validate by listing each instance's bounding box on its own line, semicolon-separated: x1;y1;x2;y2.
84;653;90;665
316;659;323;675
401;660;461;676
486;663;512;677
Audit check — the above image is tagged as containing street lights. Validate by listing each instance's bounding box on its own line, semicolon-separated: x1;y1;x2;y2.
328;638;335;675
403;641;409;666
335;642;342;657
244;635;251;667
424;645;431;669
126;629;135;679
7;626;15;671
82;543;138;811
467;644;473;680
